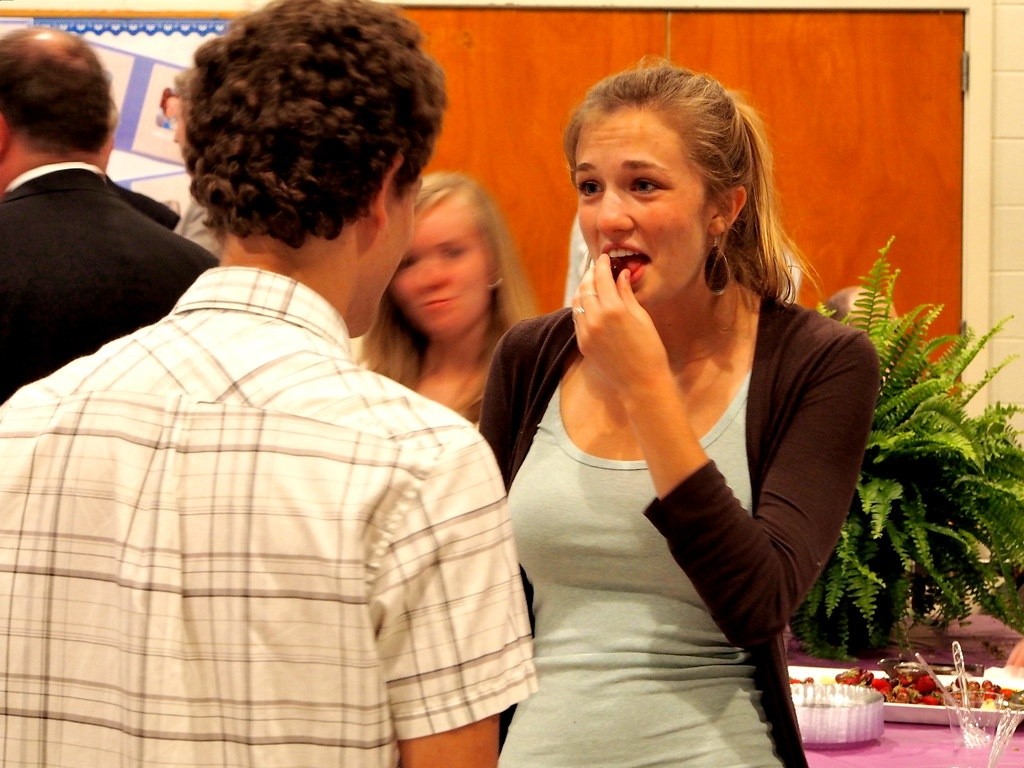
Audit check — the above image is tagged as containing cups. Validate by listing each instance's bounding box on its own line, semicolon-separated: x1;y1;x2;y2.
943;691;1003;768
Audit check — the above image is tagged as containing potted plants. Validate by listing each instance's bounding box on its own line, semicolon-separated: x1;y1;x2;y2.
784;233;1024;664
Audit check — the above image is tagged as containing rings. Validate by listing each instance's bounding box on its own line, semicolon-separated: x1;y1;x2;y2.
571;307;584;323
584;292;596;296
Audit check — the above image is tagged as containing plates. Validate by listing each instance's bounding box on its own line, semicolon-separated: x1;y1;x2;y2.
790;683;883;744
786;664;1024;728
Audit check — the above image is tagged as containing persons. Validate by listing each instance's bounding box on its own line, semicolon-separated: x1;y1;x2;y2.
476;55;883;768
0;26;372;401
0;0;538;768
361;169;529;430
828;282;900;354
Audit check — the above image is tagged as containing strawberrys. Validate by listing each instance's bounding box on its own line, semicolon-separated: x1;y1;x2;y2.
786;668;946;706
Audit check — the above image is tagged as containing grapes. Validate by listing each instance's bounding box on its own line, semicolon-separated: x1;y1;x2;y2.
610;256;625;278
946;676;1003;704
